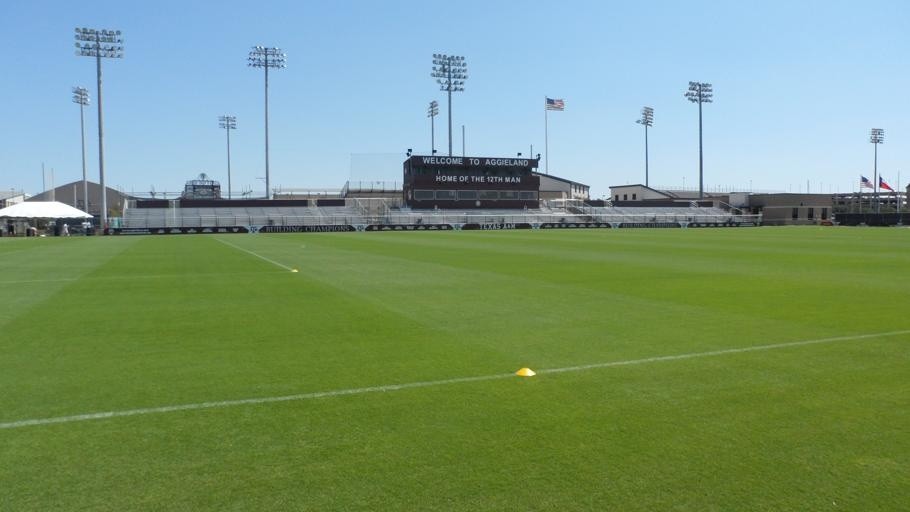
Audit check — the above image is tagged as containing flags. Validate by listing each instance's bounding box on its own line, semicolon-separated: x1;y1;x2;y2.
861;176;874;189
879;176;894;191
547;97;564;111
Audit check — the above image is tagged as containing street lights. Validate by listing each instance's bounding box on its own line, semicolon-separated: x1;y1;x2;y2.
868;129;885;199
641;106;654;186
425;99;440;159
685;81;713;199
73;84;91;217
217;110;237;200
431;53;467;159
73;26;122;229
247;46;287;203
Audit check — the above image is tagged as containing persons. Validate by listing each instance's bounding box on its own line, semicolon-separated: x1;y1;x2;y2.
7;218;93;236
0;215;95;238
